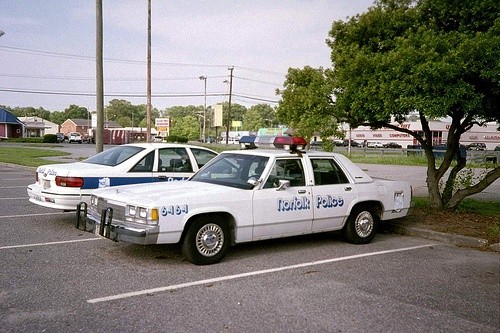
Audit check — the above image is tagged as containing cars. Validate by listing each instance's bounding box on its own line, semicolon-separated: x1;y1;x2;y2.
311;139;500;156
85;134;412;266
26;142;255;212
41;132;95;145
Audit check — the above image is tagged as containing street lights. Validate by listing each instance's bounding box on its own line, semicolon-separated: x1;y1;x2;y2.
198;75;208;143
224;65;235;145
